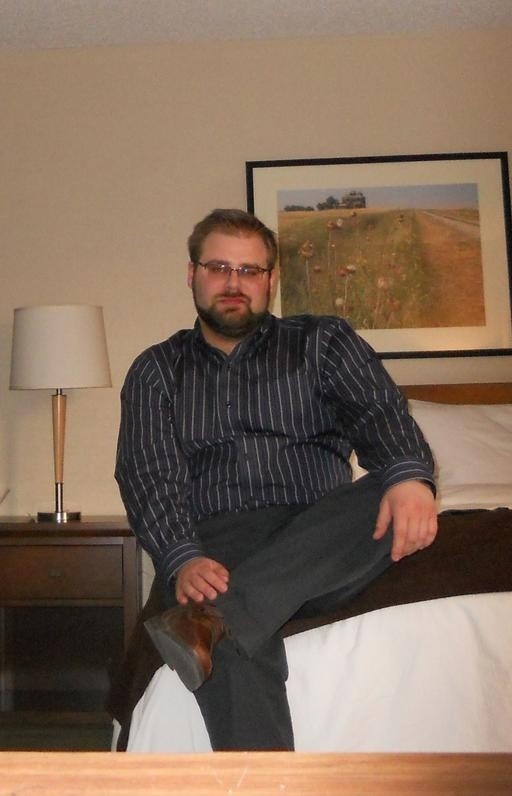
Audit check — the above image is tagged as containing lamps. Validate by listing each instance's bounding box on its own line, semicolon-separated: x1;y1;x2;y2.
7;305;112;525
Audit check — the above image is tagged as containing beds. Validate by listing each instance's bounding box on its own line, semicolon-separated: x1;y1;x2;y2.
111;380;512;752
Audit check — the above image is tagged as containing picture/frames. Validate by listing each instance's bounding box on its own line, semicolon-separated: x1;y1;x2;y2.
247;151;512;360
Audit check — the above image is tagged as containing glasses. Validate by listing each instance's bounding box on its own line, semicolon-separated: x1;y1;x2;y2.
195;259;272;281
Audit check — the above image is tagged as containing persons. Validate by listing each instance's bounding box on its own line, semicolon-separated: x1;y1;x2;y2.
108;206;442;754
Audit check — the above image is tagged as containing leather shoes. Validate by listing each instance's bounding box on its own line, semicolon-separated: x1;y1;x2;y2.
142;597;227;691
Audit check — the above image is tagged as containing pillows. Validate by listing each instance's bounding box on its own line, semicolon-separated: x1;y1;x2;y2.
407;402;510;505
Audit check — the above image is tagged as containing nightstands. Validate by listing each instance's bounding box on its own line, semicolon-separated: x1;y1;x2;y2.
0;531;143;751
